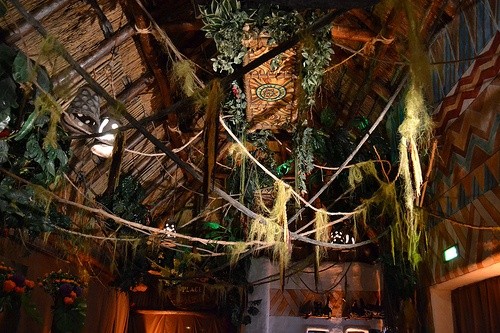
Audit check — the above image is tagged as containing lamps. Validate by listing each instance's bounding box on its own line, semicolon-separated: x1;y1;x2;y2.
329;229;357;247
164;221;175;239
66;85;118;160
